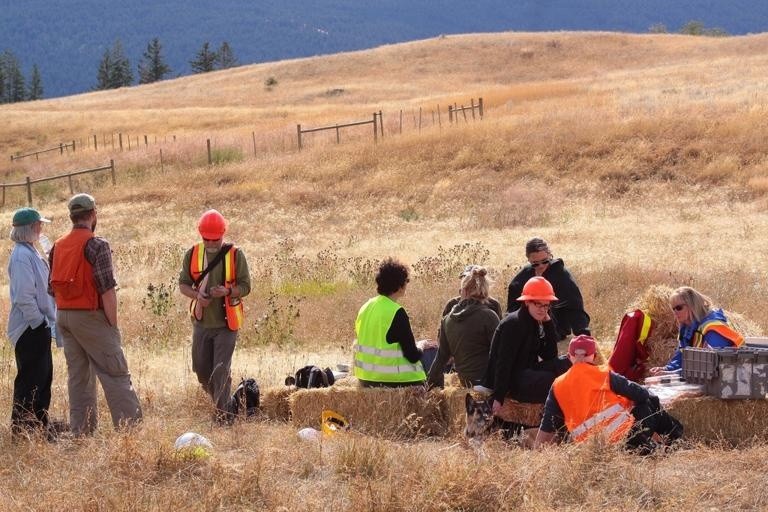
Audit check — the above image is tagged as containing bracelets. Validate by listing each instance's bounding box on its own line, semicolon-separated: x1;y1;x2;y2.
226;287;232;298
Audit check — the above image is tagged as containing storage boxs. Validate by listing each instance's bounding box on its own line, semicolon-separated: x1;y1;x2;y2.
679;344;767;402
743;337;767;349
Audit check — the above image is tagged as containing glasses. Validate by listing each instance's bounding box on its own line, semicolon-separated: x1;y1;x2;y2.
531;259;552;268
533;301;552;310
672;303;687;311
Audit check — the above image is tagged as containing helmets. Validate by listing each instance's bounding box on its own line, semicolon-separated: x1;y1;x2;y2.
197;208;227;240
320;409;349;436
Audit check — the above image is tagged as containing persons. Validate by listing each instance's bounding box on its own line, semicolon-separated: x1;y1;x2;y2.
6;207;70;445
351;257;437;387
421;266;497;386
48;193;146;438
532;334;695;453
649;285;742;379
176;208;250;419
478;276;583;399
507;240;591;354
441;269;504;320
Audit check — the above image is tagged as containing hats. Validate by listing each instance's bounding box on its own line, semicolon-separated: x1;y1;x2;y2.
515;276;559;302
67;193;96;214
568;334;597;358
11;208;52;226
